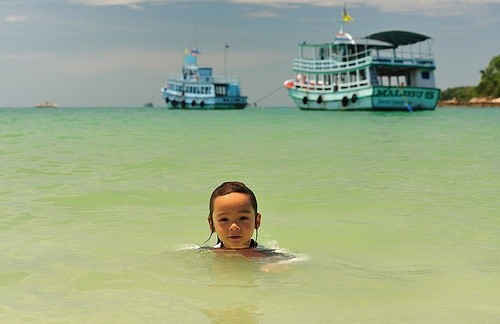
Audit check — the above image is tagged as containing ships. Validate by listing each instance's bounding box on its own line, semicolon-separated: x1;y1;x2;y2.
281;1;442;111
160;45;250;110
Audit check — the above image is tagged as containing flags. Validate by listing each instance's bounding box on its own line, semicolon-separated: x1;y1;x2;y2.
343;14;355;24
184;49;192;56
191;48;208;58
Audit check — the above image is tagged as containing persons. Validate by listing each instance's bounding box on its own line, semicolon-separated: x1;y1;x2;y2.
171;180;312;276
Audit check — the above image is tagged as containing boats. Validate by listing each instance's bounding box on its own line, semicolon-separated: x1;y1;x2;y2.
34;101;56;108
